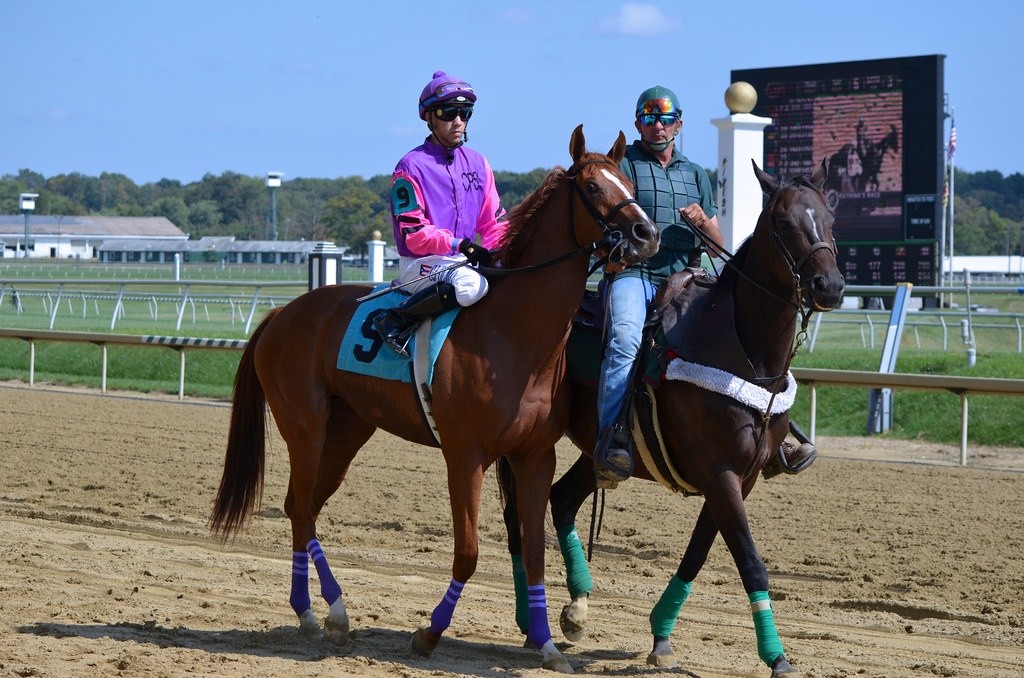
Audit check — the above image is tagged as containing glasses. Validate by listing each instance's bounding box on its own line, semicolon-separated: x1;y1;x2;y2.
429;103;472;121
638;114;679;126
434;82;475;98
640;98;676;114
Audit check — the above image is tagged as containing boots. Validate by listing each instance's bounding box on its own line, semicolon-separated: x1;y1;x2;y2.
373;281;446;359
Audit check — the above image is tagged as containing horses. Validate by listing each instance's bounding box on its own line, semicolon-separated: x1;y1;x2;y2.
207;124;847;677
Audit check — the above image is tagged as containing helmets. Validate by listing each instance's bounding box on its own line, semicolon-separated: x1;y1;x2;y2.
635;86;682;120
419;71;477;120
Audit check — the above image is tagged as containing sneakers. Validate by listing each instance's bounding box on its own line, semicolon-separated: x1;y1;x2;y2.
593;448;632;489
761;441;814;479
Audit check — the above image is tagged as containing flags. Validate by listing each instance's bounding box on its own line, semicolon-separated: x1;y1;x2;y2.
942;174;948;207
947;116;956;163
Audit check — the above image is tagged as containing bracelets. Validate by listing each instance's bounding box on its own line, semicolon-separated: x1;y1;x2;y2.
451;239;459;255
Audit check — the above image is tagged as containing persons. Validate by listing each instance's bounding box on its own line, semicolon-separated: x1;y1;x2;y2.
592;85;817;490
647;119;652;125
373;70;512;356
847;149;863;185
856;117;882;158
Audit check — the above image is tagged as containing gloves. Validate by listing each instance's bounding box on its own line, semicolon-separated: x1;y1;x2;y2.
459;238;492;268
